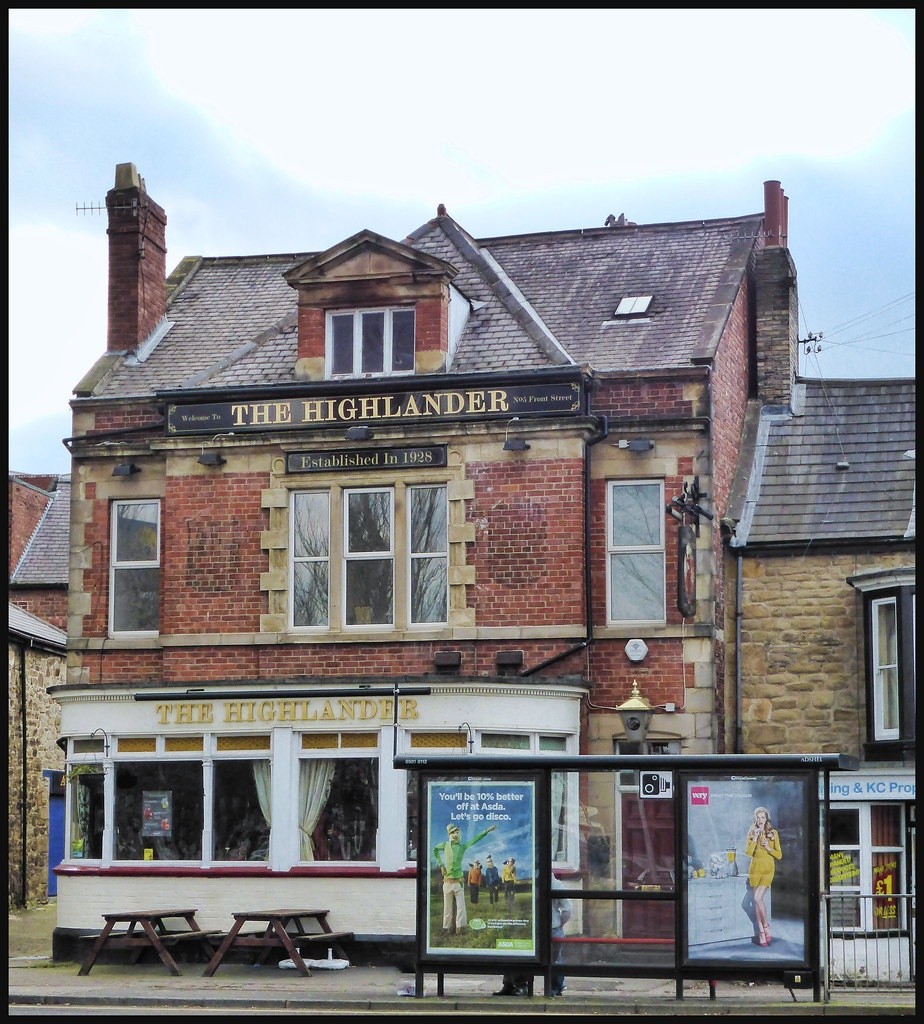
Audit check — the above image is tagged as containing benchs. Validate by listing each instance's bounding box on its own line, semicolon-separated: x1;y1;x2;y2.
202;929;271;978
546;889;716;1003
75;930;143;977
157;928;222;977
294;931;355;978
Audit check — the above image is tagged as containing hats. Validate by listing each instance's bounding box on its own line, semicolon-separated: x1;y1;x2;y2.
474;860;479;864
508;857;514;863
486;858;492;863
446;824;459;834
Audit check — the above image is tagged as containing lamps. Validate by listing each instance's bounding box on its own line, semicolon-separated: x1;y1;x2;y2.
112;464;140;477
615;678;657;748
627;439;654;452
502;439;531;452
196;453;227;467
343;426;373;441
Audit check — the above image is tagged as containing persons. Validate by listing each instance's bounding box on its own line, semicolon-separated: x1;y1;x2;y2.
551;871;572;998
493;975;530;996
432;824;516;937
746;806;782;946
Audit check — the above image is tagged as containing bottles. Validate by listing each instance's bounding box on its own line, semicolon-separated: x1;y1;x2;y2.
408;840;415;860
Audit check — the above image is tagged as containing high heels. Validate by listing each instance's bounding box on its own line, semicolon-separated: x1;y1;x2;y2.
758;932;768;946
763;924;772;944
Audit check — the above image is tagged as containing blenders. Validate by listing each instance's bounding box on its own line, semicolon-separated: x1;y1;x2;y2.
725;846;739;877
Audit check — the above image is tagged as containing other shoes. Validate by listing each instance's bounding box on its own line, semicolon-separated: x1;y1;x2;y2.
494;985;515;995
550;993;563;997
512;985;528;996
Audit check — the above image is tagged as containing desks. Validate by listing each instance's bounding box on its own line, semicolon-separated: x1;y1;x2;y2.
77;908;221;977
202;908;353;978
